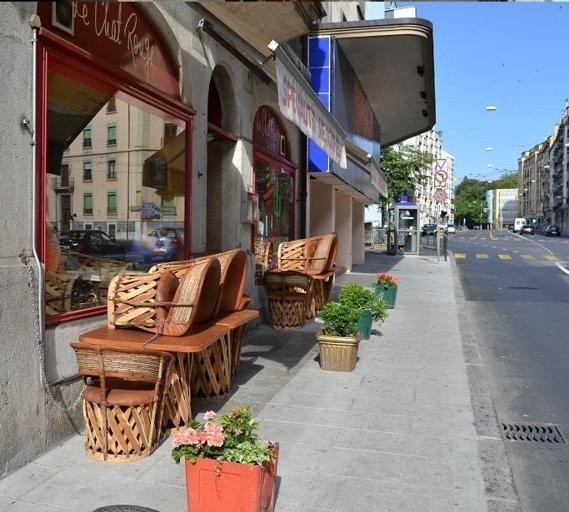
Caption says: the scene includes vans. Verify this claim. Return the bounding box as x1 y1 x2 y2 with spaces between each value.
513 217 526 233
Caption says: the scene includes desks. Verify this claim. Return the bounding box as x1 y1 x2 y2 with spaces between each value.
78 310 260 438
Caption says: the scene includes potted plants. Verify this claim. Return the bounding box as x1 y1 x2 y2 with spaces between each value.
314 301 364 372
338 282 389 341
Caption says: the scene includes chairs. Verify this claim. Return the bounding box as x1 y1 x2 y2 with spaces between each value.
254 235 282 271
106 248 248 337
69 341 168 462
45 250 134 315
263 232 345 329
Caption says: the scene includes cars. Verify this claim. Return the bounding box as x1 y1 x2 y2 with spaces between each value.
446 224 457 234
56 231 127 261
520 225 536 235
545 225 561 236
140 227 186 261
421 224 437 236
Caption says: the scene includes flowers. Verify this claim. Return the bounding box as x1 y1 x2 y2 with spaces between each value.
170 407 276 466
376 272 401 285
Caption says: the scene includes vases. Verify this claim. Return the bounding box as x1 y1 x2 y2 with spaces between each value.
371 282 398 309
184 439 280 512
